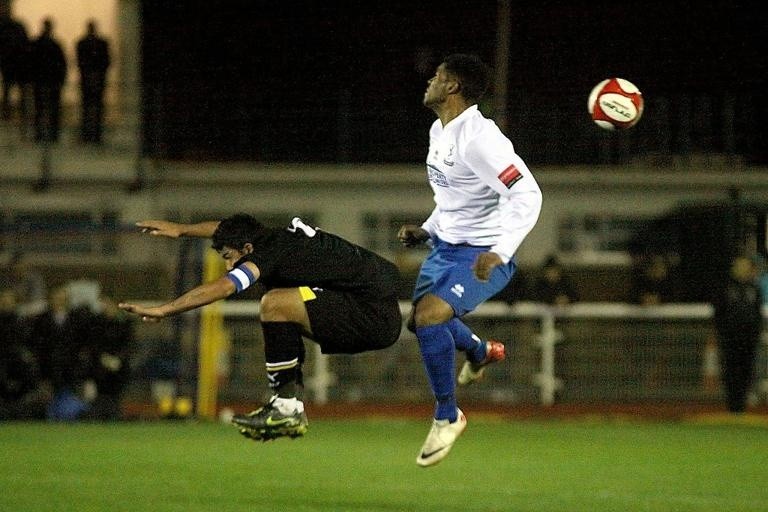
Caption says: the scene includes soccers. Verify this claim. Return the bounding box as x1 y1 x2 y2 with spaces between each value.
587 78 644 131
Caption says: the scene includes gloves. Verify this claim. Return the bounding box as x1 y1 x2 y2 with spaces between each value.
472 250 505 282
397 223 430 251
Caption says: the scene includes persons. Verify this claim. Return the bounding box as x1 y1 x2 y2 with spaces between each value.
29 16 67 146
147 310 199 420
629 204 766 413
484 258 579 389
1 249 129 423
397 51 544 472
0 0 27 125
119 214 404 443
74 17 112 146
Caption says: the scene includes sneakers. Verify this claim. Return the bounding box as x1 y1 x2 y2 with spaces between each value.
411 407 469 468
230 404 308 440
456 339 506 387
